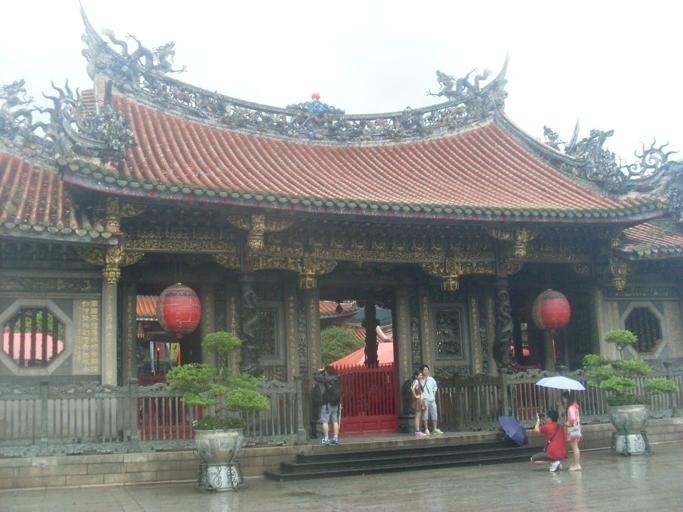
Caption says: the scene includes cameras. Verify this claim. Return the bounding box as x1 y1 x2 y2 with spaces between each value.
538 414 545 417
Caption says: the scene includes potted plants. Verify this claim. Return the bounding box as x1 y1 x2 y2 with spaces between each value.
166 330 270 493
583 329 679 456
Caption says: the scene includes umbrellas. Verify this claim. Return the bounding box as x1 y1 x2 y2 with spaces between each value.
536 375 586 404
497 415 527 446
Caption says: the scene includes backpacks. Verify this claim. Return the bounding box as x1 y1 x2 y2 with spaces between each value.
326 383 340 405
404 378 413 400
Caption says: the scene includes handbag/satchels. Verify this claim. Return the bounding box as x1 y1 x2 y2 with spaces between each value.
568 426 581 439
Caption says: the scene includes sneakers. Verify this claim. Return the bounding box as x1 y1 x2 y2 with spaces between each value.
322 436 339 444
568 463 581 471
549 461 563 471
415 431 427 437
425 430 430 436
432 429 443 435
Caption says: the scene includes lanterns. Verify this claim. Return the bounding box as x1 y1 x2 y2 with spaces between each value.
532 287 572 338
154 280 202 342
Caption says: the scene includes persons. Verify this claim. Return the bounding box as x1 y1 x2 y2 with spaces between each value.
313 365 340 445
419 364 444 436
556 391 583 471
410 370 427 438
530 410 567 472
